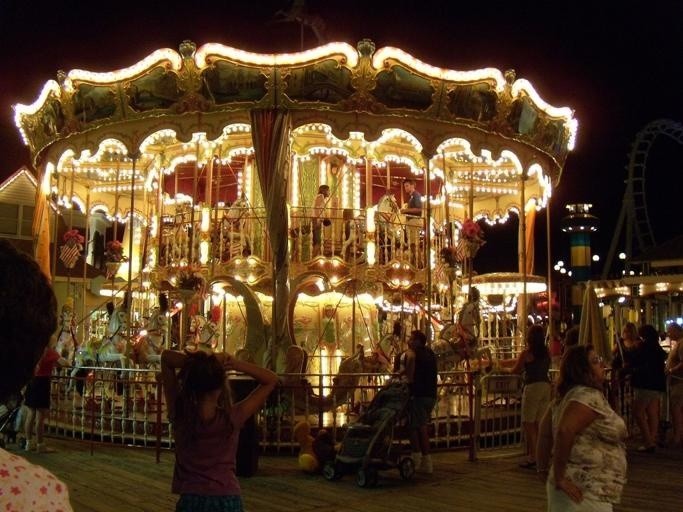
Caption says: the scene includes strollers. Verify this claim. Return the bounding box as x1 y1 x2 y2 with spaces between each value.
0 394 26 450
319 378 417 488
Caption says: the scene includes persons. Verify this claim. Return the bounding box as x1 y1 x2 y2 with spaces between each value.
400 179 423 269
312 185 330 258
161 351 279 512
539 344 628 510
394 319 438 474
1 237 73 512
502 324 551 467
527 317 682 450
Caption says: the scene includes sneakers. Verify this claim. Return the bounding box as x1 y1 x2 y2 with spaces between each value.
36 443 56 453
417 462 432 473
25 440 36 452
